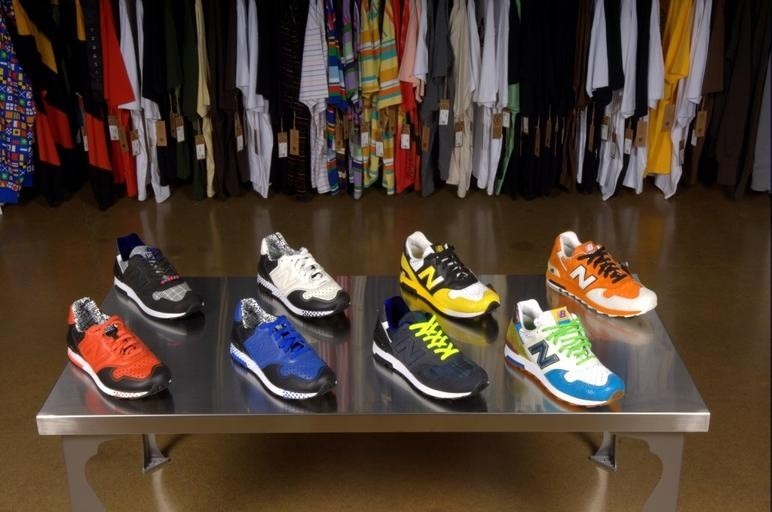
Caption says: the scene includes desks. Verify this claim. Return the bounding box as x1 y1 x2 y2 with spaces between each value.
35 276 712 472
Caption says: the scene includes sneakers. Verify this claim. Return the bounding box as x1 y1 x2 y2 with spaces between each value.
503 360 623 413
546 286 655 346
256 232 350 317
253 288 352 343
229 296 336 400
372 358 489 416
372 294 502 399
398 230 501 321
399 287 499 347
231 359 338 416
66 296 172 401
545 230 658 318
113 232 202 319
68 362 176 414
111 288 204 347
503 299 626 406
